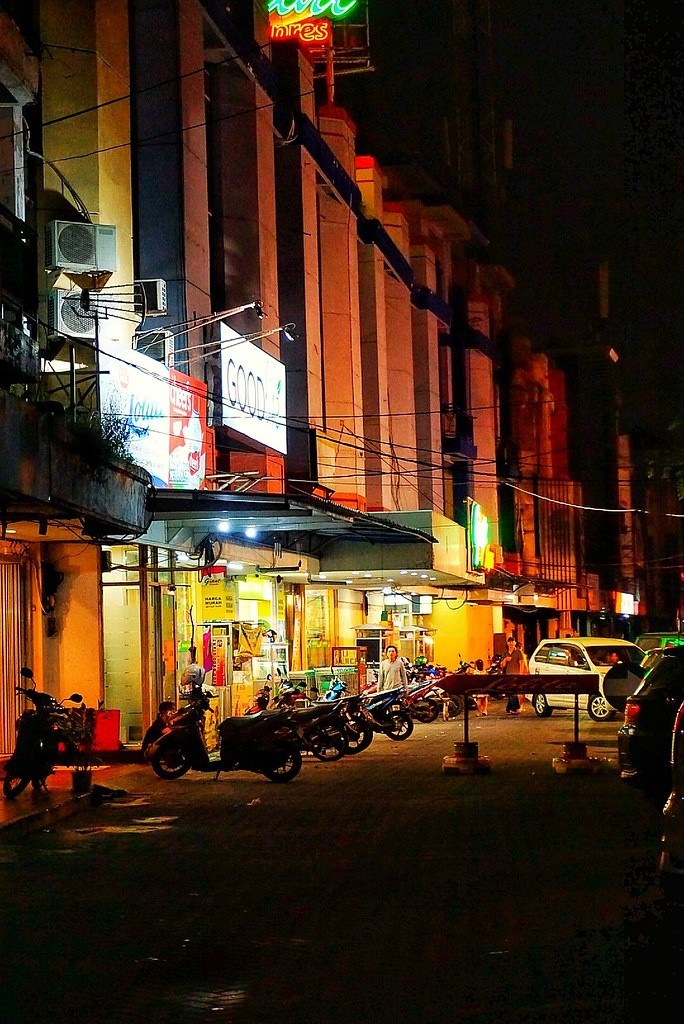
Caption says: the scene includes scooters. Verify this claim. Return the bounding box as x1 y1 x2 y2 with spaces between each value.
144 650 303 786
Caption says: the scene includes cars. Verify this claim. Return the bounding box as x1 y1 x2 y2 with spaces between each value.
636 631 684 654
615 653 684 785
656 699 683 891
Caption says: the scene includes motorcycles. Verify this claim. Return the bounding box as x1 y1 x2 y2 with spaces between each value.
2 666 88 802
233 652 502 763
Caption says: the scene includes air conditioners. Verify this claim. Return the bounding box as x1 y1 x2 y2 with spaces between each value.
43 220 119 274
46 288 123 341
134 278 168 317
132 329 175 370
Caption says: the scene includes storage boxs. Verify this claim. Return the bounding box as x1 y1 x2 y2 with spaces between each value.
253 640 290 711
331 646 369 697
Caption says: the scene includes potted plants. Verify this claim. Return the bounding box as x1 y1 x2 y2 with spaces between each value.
67 698 105 793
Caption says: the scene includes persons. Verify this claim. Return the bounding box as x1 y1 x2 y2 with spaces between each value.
607 651 619 665
514 642 529 714
472 657 490 717
498 637 525 715
378 645 408 732
140 701 177 764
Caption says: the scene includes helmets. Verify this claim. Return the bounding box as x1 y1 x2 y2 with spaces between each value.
182 665 206 687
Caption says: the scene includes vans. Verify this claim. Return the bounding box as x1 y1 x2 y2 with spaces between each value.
525 636 651 721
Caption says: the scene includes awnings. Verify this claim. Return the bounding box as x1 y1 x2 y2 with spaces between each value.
154 485 439 554
